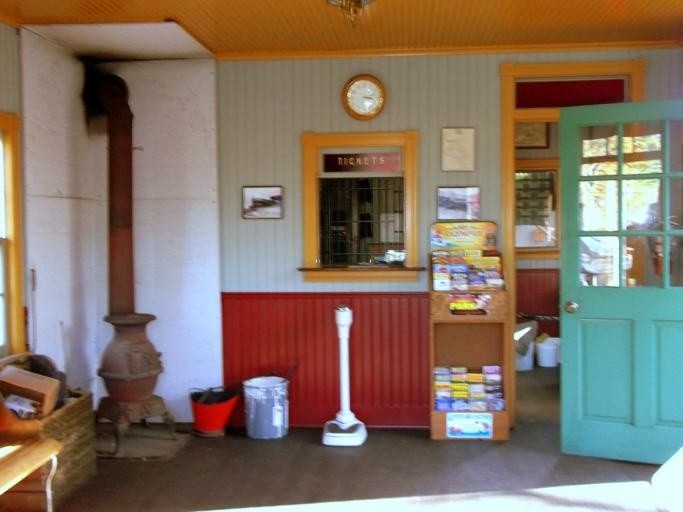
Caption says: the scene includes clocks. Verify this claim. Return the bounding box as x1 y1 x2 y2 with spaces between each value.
341 73 386 122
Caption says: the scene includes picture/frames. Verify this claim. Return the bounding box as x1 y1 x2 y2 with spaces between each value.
435 184 481 221
513 121 549 149
240 184 284 220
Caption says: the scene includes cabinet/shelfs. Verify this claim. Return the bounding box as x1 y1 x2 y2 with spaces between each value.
426 221 509 442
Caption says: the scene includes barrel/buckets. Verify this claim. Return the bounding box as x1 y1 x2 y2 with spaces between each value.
536 336 559 368
547 337 560 364
514 341 535 371
242 376 290 440
